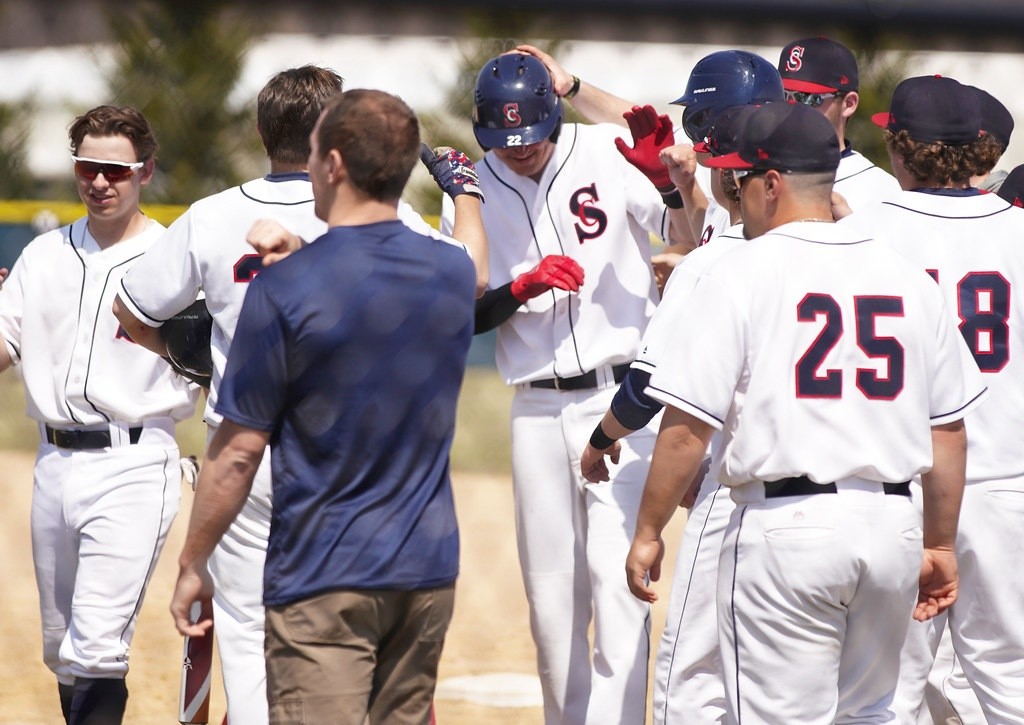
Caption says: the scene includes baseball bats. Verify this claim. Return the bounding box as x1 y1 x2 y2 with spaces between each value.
179 597 214 725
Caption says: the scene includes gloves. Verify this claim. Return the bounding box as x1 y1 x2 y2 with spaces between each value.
511 253 585 305
614 104 683 195
418 141 486 205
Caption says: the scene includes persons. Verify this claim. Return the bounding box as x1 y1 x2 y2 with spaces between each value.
504 36 900 725
112 64 490 724
626 100 990 725
836 74 1024 725
442 55 695 725
0 104 205 725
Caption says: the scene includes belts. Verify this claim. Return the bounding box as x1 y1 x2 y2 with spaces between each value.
764 479 911 499
530 361 631 390
45 424 143 450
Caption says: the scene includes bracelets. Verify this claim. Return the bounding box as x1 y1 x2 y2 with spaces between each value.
660 187 677 195
563 74 580 99
590 421 618 450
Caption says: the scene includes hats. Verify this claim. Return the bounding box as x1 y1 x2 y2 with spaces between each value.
871 74 983 146
996 163 1024 210
693 104 768 156
703 99 842 174
777 34 860 96
964 85 1015 155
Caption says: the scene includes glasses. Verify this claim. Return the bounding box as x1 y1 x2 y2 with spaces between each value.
722 167 794 194
70 153 144 182
784 89 844 108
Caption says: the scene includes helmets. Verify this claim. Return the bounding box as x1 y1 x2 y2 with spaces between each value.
470 53 564 153
664 49 786 143
162 297 214 388
